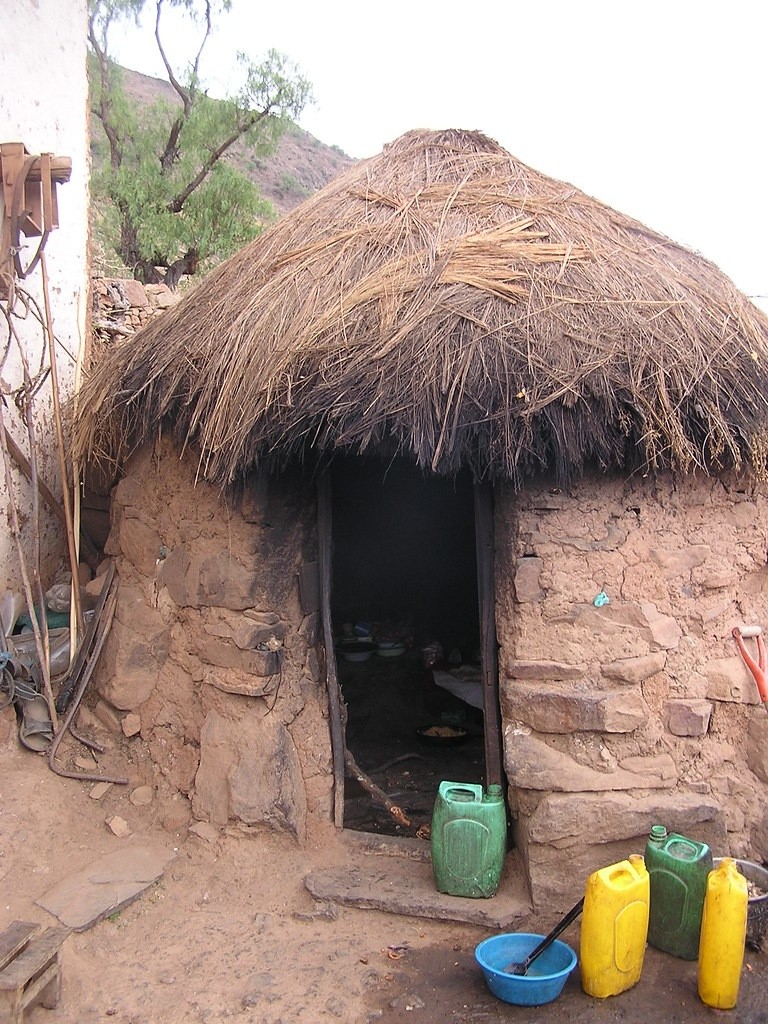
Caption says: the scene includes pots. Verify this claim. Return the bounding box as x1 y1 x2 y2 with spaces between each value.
712 856 768 944
414 721 473 747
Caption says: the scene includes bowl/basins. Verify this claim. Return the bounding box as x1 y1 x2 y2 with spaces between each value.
342 650 375 662
476 933 578 1006
378 642 408 656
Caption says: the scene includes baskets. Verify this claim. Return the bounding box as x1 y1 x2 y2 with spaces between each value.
415 722 468 747
712 858 768 945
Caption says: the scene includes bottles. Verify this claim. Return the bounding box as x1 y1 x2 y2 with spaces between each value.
579 854 650 998
45 583 99 613
431 780 507 900
697 857 748 1008
644 825 714 961
